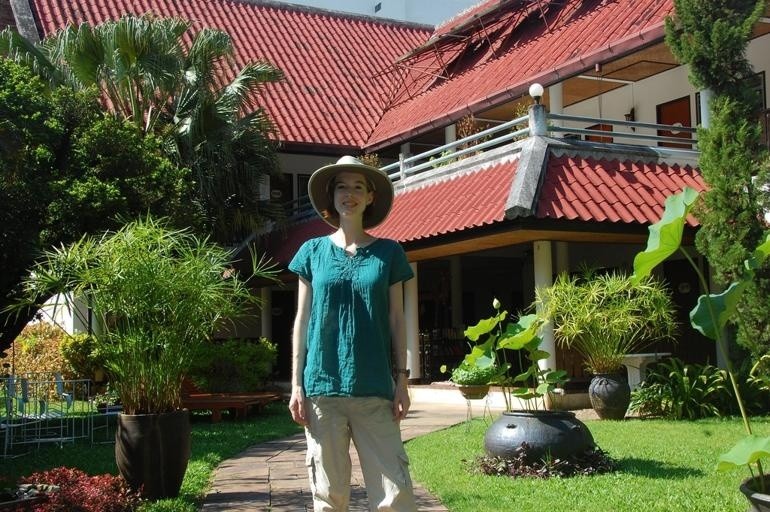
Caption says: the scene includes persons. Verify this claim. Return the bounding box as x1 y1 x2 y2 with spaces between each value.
286 156 421 510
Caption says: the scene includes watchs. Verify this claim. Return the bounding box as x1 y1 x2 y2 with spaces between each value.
396 367 410 380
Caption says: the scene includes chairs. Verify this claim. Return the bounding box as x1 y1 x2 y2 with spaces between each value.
0 372 123 459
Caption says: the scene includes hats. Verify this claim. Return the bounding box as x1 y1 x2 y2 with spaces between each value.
308 155 394 229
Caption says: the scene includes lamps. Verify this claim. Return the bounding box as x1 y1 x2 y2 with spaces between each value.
528 83 544 106
624 108 635 132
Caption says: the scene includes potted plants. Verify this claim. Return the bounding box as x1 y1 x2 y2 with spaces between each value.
0 211 284 500
630 185 770 512
649 357 727 419
451 349 507 399
464 298 600 473
522 267 680 419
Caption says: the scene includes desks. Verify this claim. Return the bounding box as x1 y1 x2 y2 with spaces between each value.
420 339 456 381
